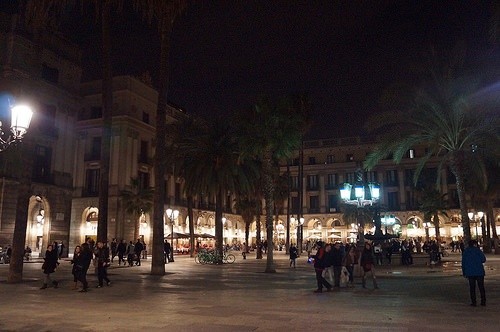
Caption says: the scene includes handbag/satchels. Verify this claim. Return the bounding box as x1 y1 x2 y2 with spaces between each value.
341 266 350 283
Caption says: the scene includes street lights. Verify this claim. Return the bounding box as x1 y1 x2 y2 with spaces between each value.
338 183 382 268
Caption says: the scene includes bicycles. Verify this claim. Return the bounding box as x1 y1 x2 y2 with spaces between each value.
194 245 236 264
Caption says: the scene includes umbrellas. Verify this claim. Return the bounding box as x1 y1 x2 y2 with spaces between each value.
165 232 215 239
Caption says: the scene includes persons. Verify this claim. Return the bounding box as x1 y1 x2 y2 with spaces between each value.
306 239 380 293
39 245 59 289
450 236 483 252
70 237 111 292
164 240 171 264
289 243 301 269
110 238 146 267
25 246 31 261
462 240 487 307
373 237 448 266
52 240 65 271
198 239 286 260
0 244 12 264
